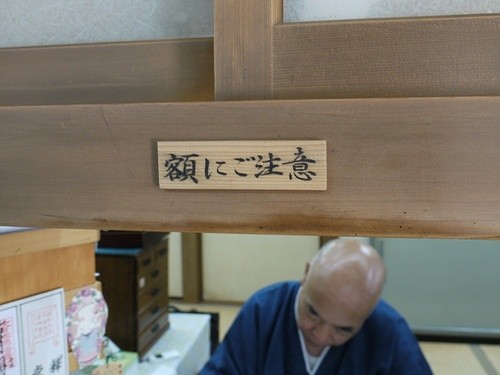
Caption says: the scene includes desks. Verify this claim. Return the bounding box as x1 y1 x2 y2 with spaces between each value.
120 311 219 375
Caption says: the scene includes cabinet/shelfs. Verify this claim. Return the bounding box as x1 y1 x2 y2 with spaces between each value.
97 239 169 356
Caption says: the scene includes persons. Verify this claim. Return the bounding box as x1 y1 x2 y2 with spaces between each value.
196 237 437 375
